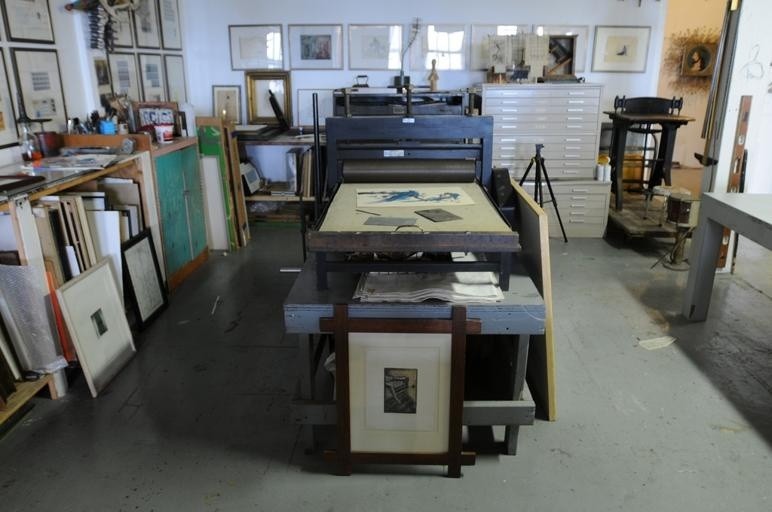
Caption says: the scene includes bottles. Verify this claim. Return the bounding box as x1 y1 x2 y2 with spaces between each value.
19 121 43 162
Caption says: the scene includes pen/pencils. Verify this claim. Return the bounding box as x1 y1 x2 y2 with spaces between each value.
105 110 114 121
90 110 99 128
356 209 380 217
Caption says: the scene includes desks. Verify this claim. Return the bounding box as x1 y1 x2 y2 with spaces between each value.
1 153 151 439
230 126 329 251
685 190 771 323
604 108 695 212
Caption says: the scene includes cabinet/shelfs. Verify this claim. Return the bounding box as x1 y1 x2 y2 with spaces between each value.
156 140 213 281
519 178 610 239
483 85 601 181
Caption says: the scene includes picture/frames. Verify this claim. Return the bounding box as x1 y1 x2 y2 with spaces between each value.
320 299 480 479
296 88 335 128
680 41 719 77
106 0 189 108
245 70 292 124
51 226 169 402
0 0 68 151
211 85 243 127
